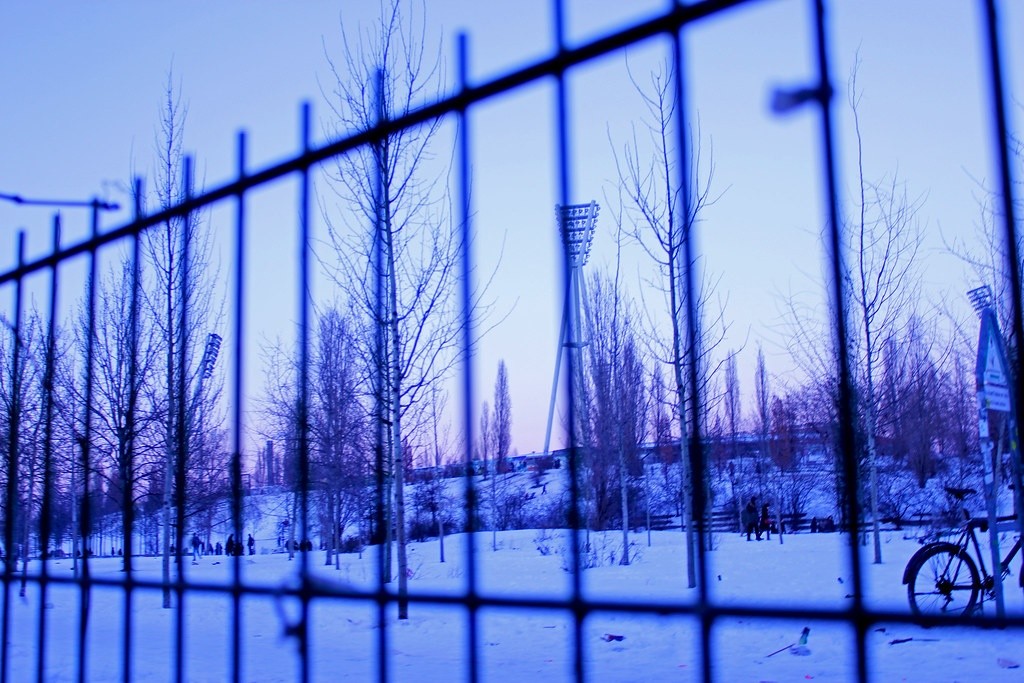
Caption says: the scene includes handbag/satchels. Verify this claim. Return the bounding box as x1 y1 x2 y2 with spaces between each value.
765 519 772 524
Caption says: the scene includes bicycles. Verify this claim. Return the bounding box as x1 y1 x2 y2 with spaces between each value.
902 485 1024 628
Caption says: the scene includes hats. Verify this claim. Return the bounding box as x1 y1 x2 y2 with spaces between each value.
750 496 758 504
765 503 772 507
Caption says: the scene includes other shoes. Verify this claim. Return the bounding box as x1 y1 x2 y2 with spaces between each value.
747 538 753 541
758 538 764 541
756 537 762 540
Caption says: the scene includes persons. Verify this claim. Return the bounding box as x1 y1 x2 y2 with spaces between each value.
192 531 255 561
170 544 175 555
285 537 313 551
740 496 777 541
809 517 817 533
